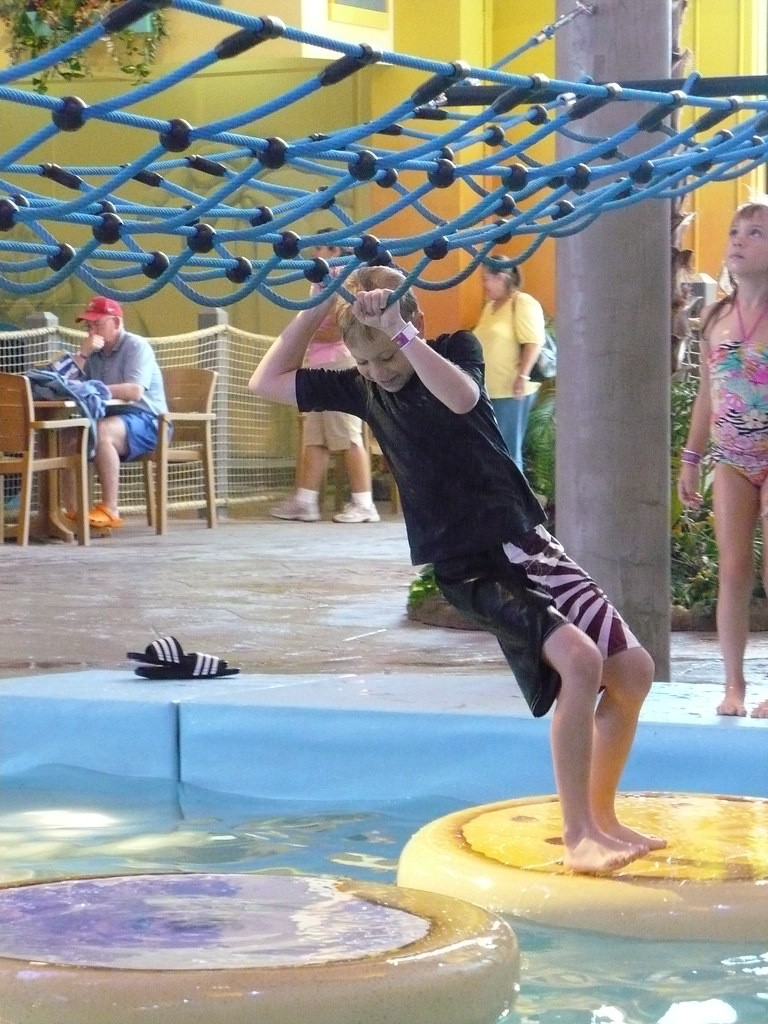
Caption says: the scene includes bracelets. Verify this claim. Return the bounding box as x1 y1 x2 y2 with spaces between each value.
391 321 419 349
681 450 703 466
76 351 89 359
518 373 531 380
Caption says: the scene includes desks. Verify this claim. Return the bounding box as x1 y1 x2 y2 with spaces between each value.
4 398 134 544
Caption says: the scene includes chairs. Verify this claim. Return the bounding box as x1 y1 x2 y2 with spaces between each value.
87 365 220 534
0 373 91 546
294 414 402 515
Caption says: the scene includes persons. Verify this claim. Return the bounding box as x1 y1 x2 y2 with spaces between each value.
679 205 768 718
247 259 667 872
271 228 381 523
473 255 544 473
62 298 175 528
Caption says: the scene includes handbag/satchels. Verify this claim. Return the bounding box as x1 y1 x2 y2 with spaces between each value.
512 291 558 382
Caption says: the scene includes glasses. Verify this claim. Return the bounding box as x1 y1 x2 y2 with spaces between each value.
81 317 116 331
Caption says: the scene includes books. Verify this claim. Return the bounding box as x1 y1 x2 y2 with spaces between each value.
36 354 86 380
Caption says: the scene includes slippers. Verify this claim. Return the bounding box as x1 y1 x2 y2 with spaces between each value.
134 651 241 680
87 504 124 528
126 635 193 667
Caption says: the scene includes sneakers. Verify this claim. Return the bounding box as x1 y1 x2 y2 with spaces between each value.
267 496 321 521
331 501 381 523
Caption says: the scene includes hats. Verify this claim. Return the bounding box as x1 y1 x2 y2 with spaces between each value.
74 294 123 323
